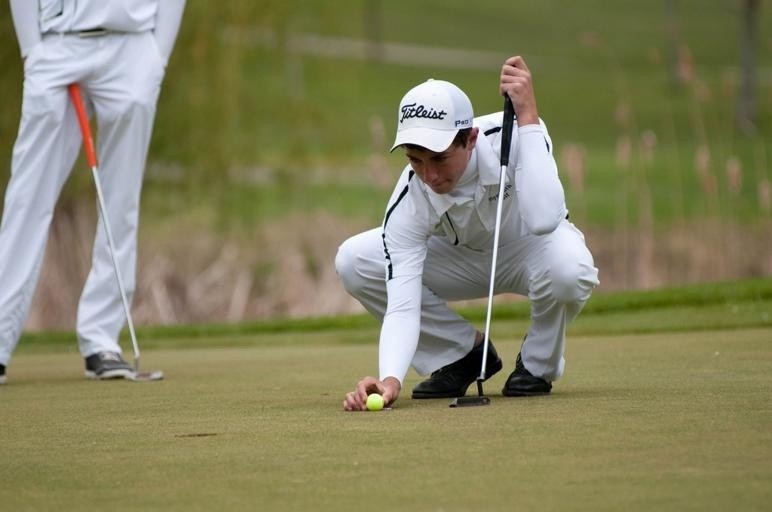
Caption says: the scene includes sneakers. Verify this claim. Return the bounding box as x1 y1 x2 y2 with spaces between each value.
85 351 136 382
0 364 10 388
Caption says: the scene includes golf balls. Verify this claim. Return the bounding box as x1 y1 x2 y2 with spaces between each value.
367 394 384 411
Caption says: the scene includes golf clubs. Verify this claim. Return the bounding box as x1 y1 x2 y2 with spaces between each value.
69 83 165 380
448 93 513 410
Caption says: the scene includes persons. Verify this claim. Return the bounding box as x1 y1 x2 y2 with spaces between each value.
336 56 598 411
2 0 189 379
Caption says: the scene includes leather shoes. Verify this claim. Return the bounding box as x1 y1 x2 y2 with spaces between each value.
411 331 504 401
501 332 554 398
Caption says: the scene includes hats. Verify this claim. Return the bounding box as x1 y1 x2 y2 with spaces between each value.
389 77 475 155
